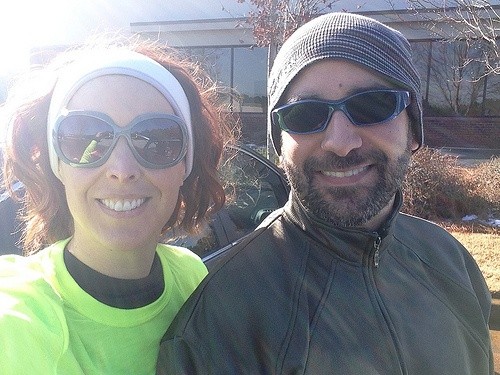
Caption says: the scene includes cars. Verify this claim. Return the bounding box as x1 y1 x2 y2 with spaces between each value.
0 132 291 265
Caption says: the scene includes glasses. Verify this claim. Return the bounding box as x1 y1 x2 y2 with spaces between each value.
271 90 412 135
51 110 190 170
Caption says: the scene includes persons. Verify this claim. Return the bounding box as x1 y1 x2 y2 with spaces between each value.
155 11 493 375
0 31 243 375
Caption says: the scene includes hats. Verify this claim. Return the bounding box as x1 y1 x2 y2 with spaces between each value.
267 13 424 160
